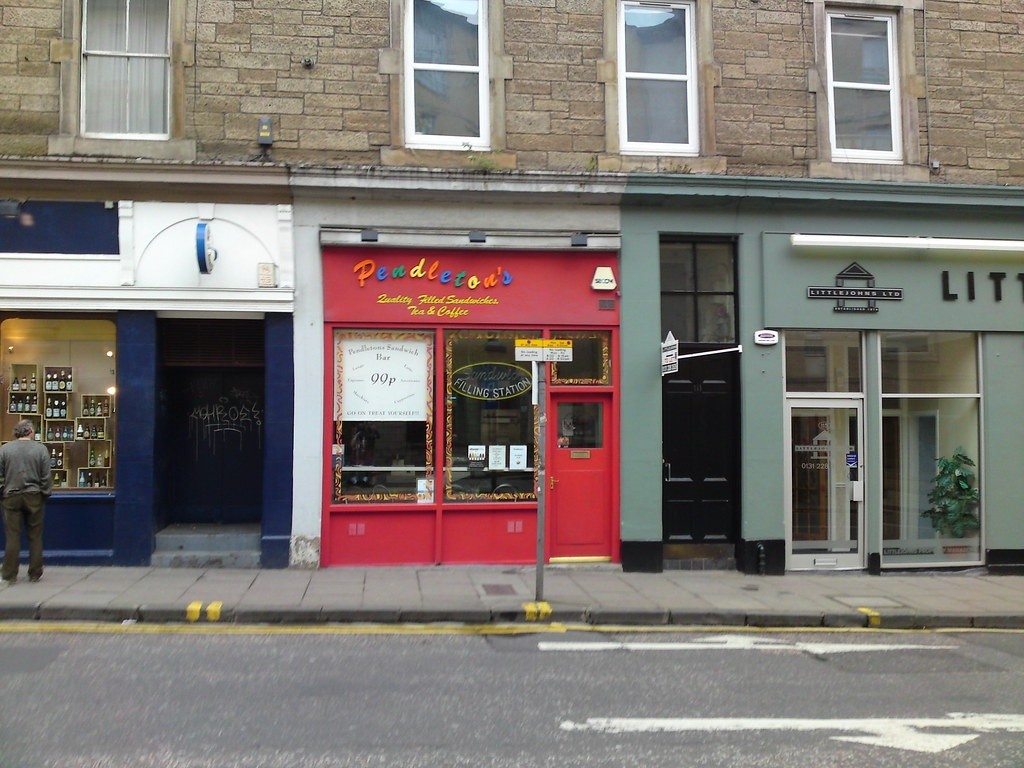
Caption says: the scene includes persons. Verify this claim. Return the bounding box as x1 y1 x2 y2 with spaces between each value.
0 419 53 583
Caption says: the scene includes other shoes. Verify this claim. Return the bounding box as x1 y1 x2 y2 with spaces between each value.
30 573 39 582
7 578 17 584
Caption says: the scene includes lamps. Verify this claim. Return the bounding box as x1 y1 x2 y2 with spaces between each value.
257 116 274 145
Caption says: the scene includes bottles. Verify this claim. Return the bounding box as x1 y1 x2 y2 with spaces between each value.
45 369 72 391
90 447 109 467
77 423 104 439
10 393 37 413
79 471 106 487
53 472 66 487
13 373 36 391
34 421 41 441
349 429 373 487
83 398 109 416
46 396 66 418
48 424 73 441
50 448 62 469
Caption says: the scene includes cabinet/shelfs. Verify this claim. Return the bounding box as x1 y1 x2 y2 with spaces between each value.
7 361 116 491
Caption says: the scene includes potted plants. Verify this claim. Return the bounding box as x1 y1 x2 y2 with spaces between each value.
922 445 981 558
